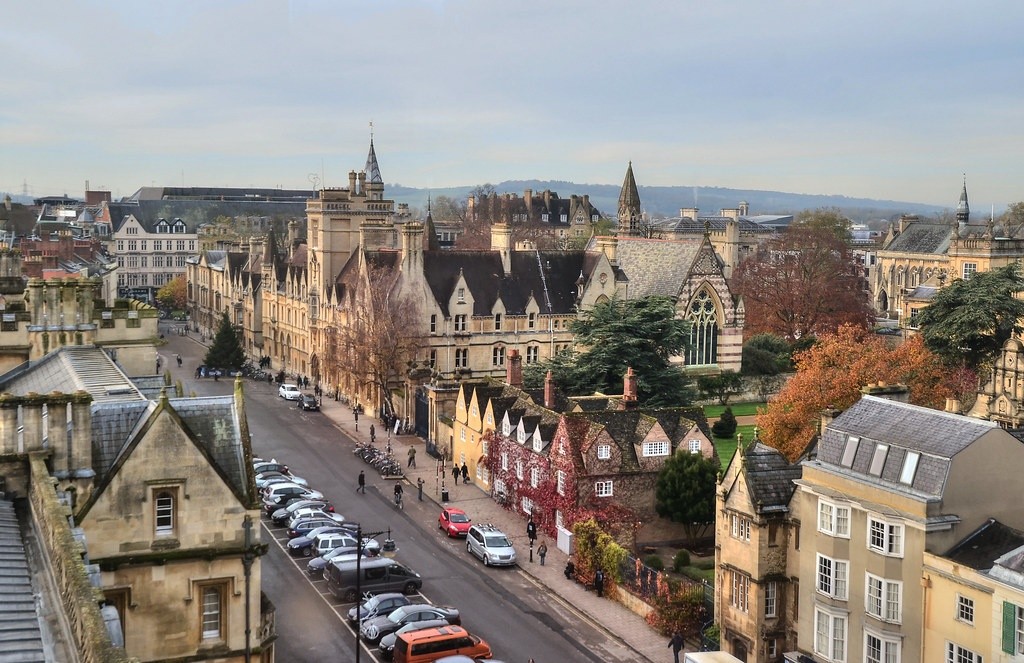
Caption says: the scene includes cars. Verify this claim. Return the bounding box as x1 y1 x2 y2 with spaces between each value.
253 455 423 603
347 592 506 663
296 393 320 412
278 384 302 401
438 508 472 538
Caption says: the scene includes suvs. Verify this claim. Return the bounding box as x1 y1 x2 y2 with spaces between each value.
466 523 517 568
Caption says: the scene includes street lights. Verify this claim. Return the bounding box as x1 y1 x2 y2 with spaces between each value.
355 523 400 663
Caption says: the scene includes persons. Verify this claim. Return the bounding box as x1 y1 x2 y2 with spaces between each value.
157 320 202 379
460 462 468 484
259 355 319 397
357 470 366 494
668 631 684 663
536 540 547 566
417 477 426 501
370 413 395 442
595 570 604 597
563 559 574 579
407 445 417 468
451 463 460 485
394 481 403 507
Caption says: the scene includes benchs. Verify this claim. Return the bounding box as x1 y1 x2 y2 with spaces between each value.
571 565 595 592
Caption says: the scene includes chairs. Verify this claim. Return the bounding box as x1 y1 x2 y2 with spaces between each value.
460 515 465 520
426 645 432 652
415 645 422 652
452 515 456 520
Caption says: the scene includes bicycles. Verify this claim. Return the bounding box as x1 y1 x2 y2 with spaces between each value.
247 369 267 381
352 441 402 476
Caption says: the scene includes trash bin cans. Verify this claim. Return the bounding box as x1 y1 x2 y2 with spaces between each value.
442 491 449 501
